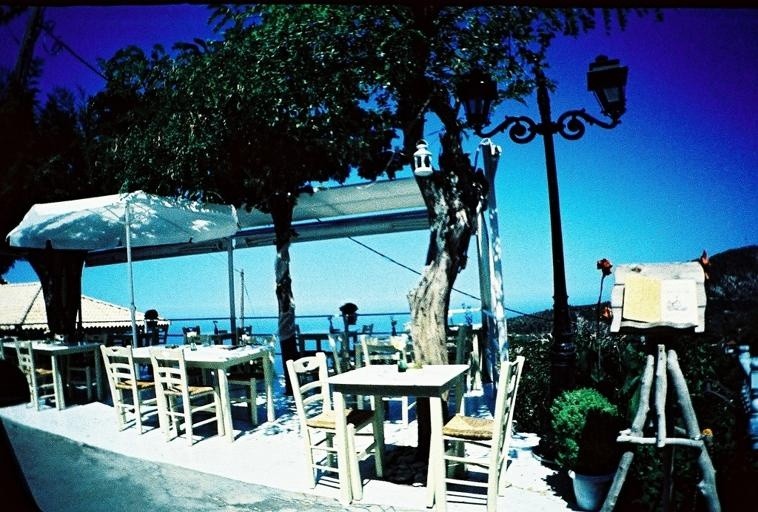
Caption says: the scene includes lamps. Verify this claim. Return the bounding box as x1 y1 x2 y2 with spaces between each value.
413 139 433 176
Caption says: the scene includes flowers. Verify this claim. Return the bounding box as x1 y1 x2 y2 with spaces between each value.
588 256 616 382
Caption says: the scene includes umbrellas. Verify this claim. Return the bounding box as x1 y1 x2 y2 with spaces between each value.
4 188 243 417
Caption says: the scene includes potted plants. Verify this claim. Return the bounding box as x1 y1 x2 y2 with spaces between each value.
549 383 628 510
